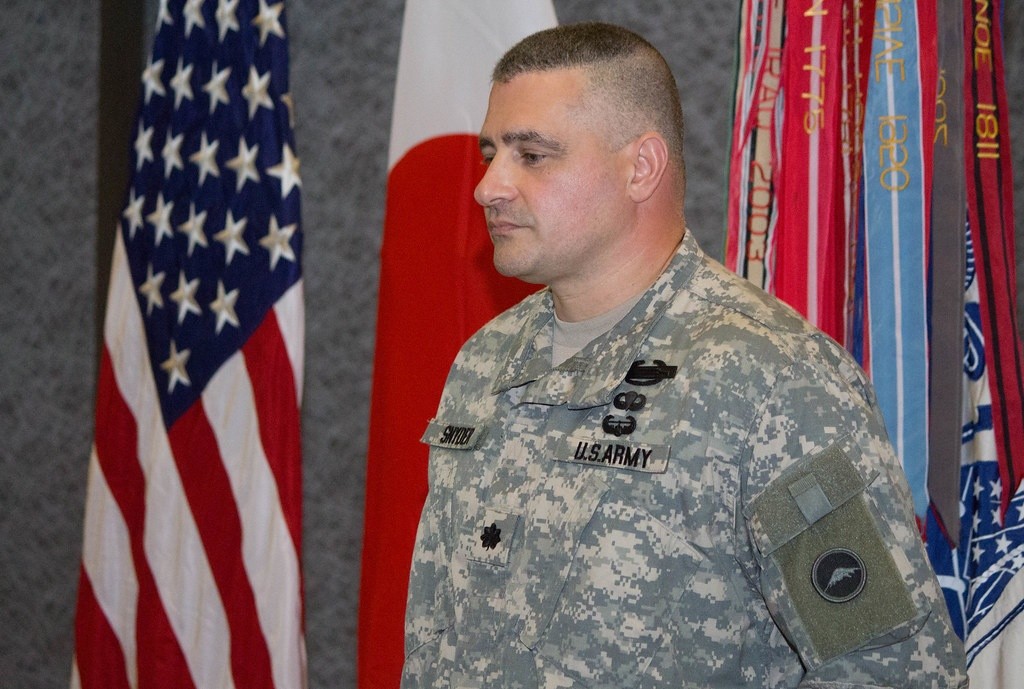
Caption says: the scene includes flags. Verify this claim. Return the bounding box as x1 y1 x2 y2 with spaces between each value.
357 0 559 688
70 1 306 688
724 0 1024 688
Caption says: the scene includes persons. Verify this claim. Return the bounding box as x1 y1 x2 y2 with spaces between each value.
400 22 969 688
